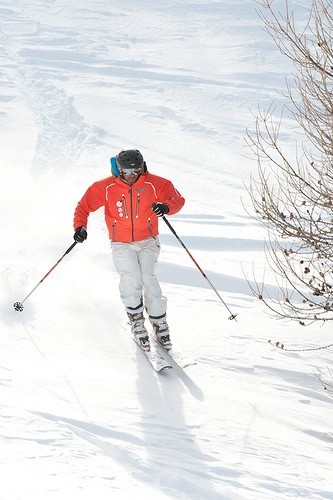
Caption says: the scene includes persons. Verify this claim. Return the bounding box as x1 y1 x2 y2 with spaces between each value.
74 149 185 350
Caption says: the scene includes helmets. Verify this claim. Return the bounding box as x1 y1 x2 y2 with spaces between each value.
117 150 144 169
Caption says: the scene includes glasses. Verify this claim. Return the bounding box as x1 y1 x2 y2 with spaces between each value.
119 168 142 176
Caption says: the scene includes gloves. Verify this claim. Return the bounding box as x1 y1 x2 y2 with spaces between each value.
151 201 169 217
73 227 87 243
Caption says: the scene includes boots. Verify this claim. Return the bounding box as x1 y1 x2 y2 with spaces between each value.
126 305 150 351
149 311 173 352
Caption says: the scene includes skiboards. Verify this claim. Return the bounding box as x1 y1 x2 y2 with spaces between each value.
120 319 173 373
150 328 198 369
